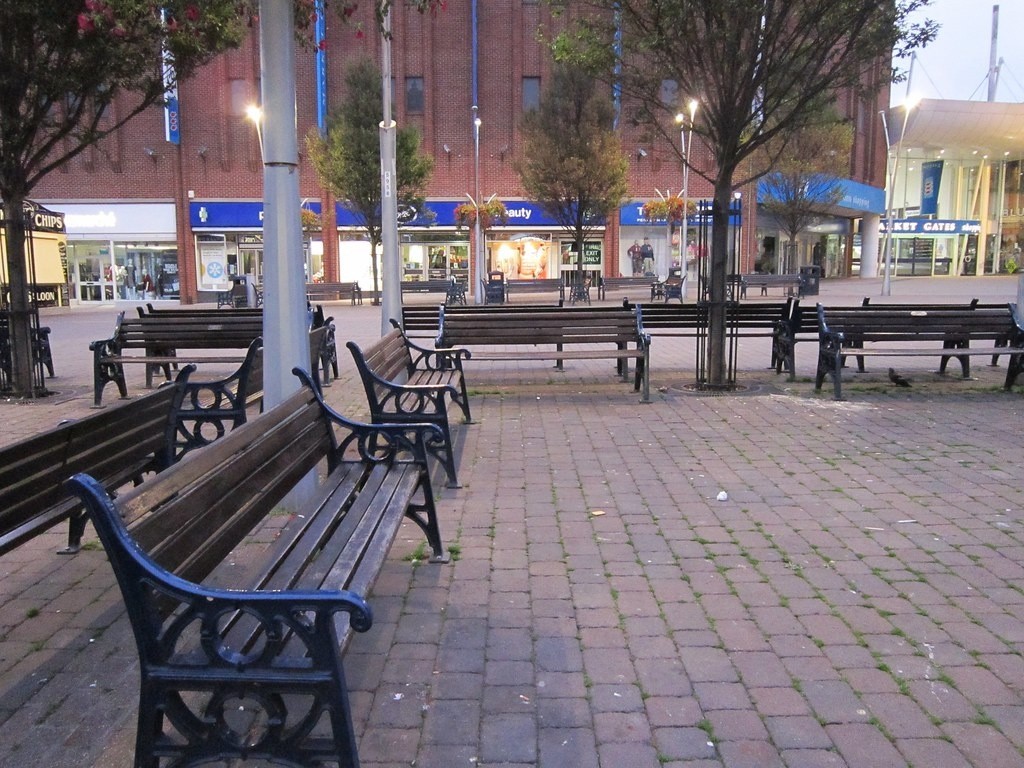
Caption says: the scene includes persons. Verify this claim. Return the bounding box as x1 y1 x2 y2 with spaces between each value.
125 258 163 300
628 237 655 276
813 242 823 274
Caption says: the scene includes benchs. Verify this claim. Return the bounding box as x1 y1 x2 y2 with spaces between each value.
0 276 1024 768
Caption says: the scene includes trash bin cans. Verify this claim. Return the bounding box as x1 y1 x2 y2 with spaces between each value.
667 266 681 279
487 269 505 305
231 274 256 309
799 265 821 296
